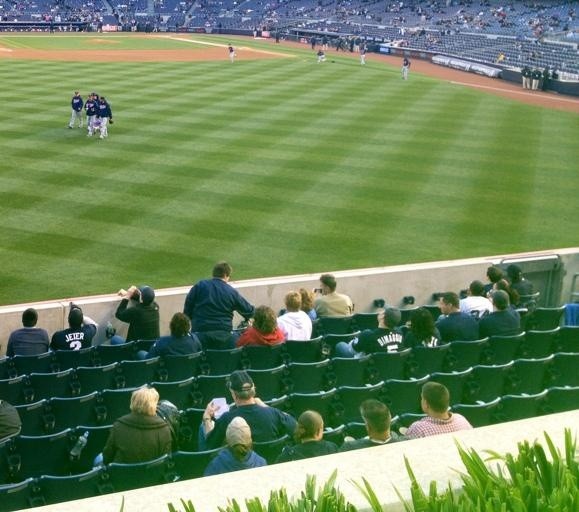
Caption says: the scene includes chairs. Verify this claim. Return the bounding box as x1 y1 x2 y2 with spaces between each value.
0 0 579 89
0 285 579 511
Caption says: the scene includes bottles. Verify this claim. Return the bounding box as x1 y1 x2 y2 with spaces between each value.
69 430 90 457
106 321 114 334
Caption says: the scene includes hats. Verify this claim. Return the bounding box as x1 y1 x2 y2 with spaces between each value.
68 305 83 326
226 369 254 392
225 416 252 448
138 285 155 303
503 264 523 277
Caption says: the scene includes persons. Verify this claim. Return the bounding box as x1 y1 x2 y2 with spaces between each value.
204 416 266 476
0 0 579 90
110 285 160 344
341 399 409 452
235 265 537 358
407 382 473 438
136 312 202 360
6 308 49 357
198 371 297 452
68 92 113 139
275 411 339 463
50 308 99 351
184 263 254 349
103 387 171 465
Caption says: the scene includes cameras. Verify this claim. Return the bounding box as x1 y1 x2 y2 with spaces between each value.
314 288 322 294
